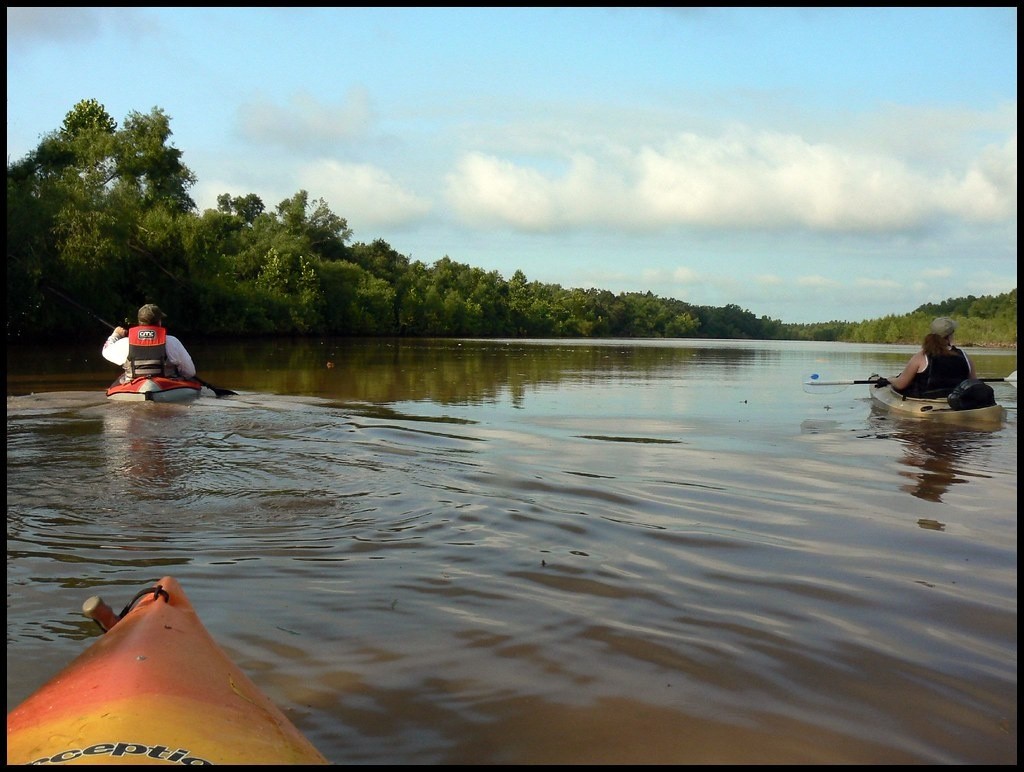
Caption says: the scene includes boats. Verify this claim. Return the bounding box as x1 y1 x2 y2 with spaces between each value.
106 375 203 402
869 371 1008 425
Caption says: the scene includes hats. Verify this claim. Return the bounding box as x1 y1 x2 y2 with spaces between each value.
138 304 167 324
931 317 957 337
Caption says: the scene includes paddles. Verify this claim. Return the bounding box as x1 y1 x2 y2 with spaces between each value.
45 285 239 398
801 368 1017 396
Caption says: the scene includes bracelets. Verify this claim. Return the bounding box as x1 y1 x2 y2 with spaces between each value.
887 375 897 381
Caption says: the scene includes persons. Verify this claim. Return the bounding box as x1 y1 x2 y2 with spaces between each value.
887 317 977 399
102 304 197 383
